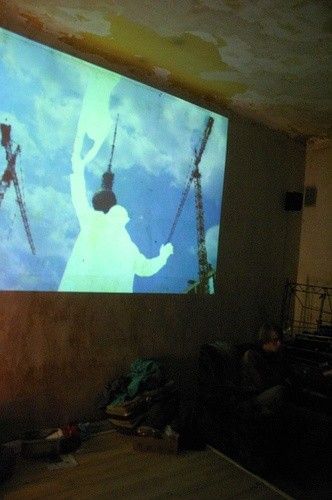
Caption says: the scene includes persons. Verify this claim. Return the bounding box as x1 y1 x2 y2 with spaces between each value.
55 156 174 293
73 57 121 171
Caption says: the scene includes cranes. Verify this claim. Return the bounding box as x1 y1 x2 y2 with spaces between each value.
0 120 39 258
164 115 216 296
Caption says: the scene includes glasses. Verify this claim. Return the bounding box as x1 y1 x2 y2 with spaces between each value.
268 336 283 343
